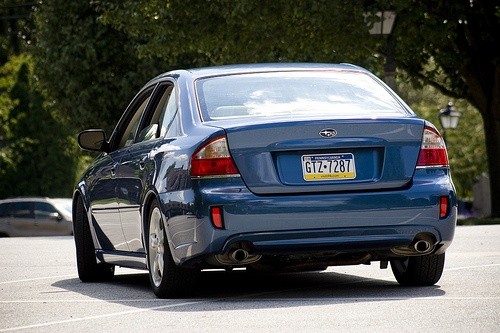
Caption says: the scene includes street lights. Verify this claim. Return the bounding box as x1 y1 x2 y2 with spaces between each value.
438 102 462 146
361 10 397 36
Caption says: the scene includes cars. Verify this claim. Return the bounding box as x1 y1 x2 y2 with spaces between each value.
71 62 457 299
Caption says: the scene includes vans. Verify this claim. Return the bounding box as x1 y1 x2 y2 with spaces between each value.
0 198 73 238
